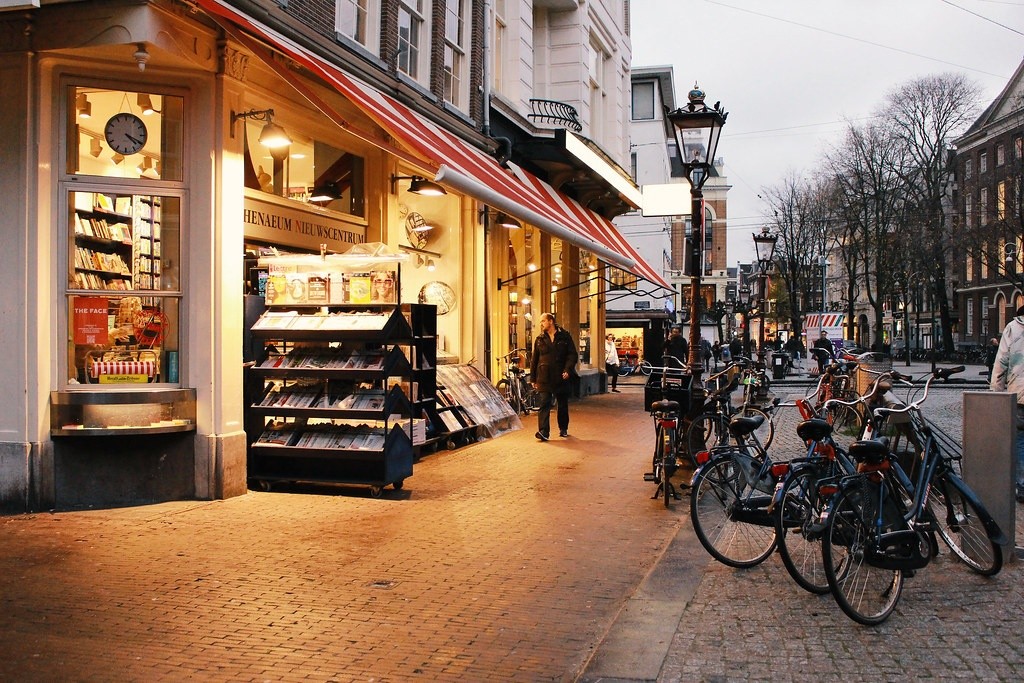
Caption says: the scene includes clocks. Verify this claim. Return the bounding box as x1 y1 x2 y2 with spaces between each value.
105 113 148 154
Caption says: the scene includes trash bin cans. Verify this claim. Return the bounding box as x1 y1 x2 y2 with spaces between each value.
721 344 732 361
854 352 892 436
766 348 777 369
771 353 789 379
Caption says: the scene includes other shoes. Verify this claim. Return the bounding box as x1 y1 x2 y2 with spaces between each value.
535 431 548 441
1015 481 1024 504
612 389 621 392
562 431 567 437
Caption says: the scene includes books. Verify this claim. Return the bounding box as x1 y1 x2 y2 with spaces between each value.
257 272 388 450
73 192 160 307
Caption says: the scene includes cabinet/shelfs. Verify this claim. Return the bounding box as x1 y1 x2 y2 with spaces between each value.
70 191 163 309
244 302 442 494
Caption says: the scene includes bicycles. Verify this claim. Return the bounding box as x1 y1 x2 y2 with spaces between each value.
617 353 654 377
810 367 1008 626
496 348 558 418
642 337 1000 596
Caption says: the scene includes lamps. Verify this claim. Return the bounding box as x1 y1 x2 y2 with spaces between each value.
478 208 522 228
230 108 293 148
390 174 447 196
307 188 335 201
134 43 151 72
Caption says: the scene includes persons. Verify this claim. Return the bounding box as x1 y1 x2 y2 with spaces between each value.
814 331 834 372
990 315 1024 506
664 328 688 368
372 273 394 303
712 341 721 370
702 338 712 371
605 334 621 393
729 337 742 361
530 313 578 441
767 336 794 351
986 338 999 383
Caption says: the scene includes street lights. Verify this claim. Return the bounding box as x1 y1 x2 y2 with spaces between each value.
738 282 753 385
662 78 729 458
751 223 779 402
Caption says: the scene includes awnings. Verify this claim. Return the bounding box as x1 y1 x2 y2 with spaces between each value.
200 0 679 294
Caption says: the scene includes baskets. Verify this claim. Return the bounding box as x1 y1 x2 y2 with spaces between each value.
644 371 694 415
